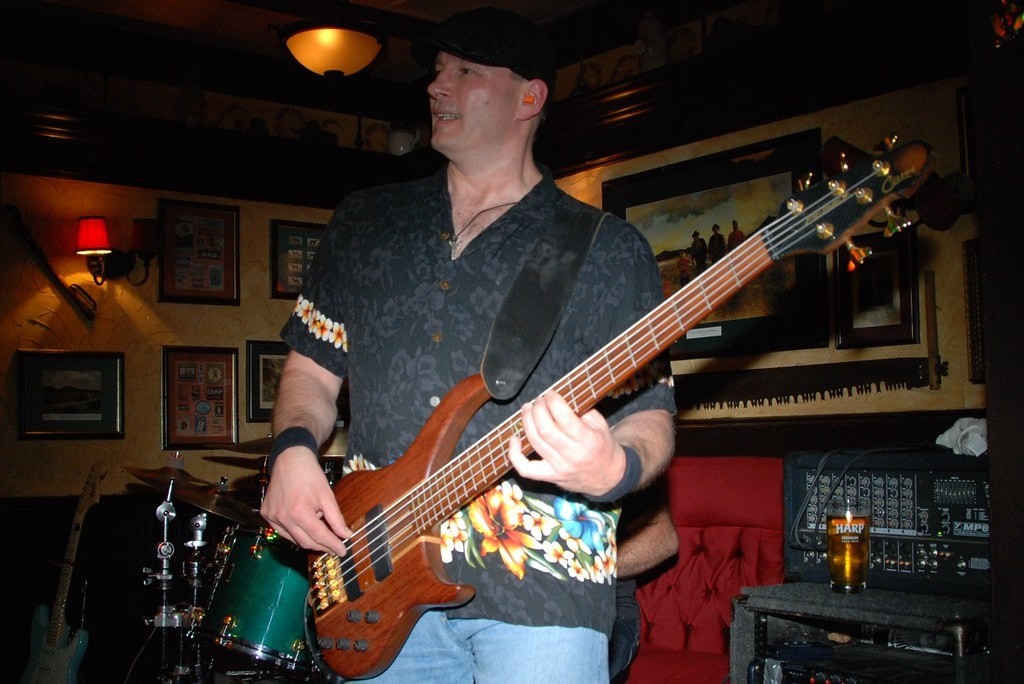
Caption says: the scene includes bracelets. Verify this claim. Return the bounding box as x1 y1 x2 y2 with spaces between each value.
581 446 640 505
267 426 319 477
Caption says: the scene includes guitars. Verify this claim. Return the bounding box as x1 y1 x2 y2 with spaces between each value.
18 460 109 684
307 133 938 681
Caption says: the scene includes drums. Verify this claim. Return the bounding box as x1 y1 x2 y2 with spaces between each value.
186 527 315 677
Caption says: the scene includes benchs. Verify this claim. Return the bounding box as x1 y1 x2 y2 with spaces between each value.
617 455 788 684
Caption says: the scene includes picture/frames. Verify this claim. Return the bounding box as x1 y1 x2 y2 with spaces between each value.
245 339 350 424
269 218 328 300
157 198 241 306
602 127 830 360
833 228 921 350
16 349 125 440
160 345 239 451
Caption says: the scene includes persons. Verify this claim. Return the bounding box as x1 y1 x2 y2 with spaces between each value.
257 7 677 684
606 486 678 680
678 219 746 279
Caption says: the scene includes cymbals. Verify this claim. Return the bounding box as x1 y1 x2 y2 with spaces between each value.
133 473 260 524
195 438 274 455
121 466 214 485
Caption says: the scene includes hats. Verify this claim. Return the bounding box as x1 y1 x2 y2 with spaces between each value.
410 7 548 84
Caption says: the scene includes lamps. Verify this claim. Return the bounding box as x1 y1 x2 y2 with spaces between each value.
72 215 163 287
264 14 388 78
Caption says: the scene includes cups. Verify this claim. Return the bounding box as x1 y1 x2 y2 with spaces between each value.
825 497 873 594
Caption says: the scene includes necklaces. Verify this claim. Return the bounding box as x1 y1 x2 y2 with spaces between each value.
448 201 519 247
